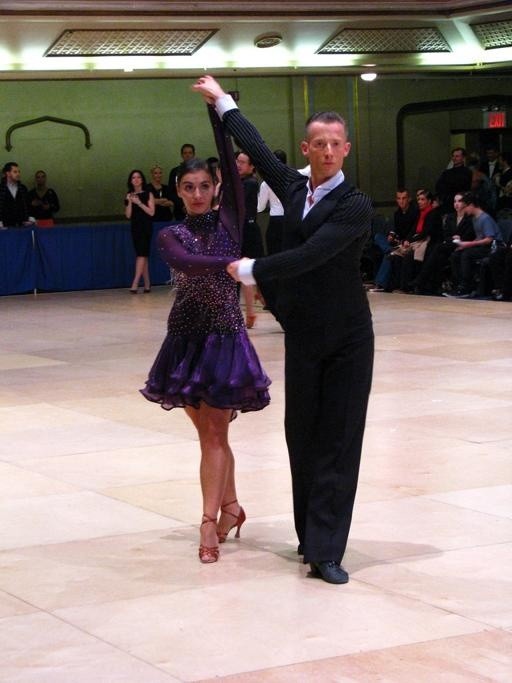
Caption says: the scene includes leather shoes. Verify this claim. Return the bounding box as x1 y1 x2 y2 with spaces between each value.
129 282 153 293
308 554 352 584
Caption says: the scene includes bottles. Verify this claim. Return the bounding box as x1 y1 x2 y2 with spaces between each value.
490 239 498 254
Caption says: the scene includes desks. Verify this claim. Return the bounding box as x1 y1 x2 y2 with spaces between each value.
1 220 178 294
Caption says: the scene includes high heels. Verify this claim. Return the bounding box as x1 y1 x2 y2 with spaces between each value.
197 513 221 563
216 499 246 542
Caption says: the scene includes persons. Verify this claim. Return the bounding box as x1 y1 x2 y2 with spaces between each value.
124 170 156 293
192 73 374 584
147 143 287 328
28 170 60 227
139 83 273 563
367 145 511 301
0 162 34 230
